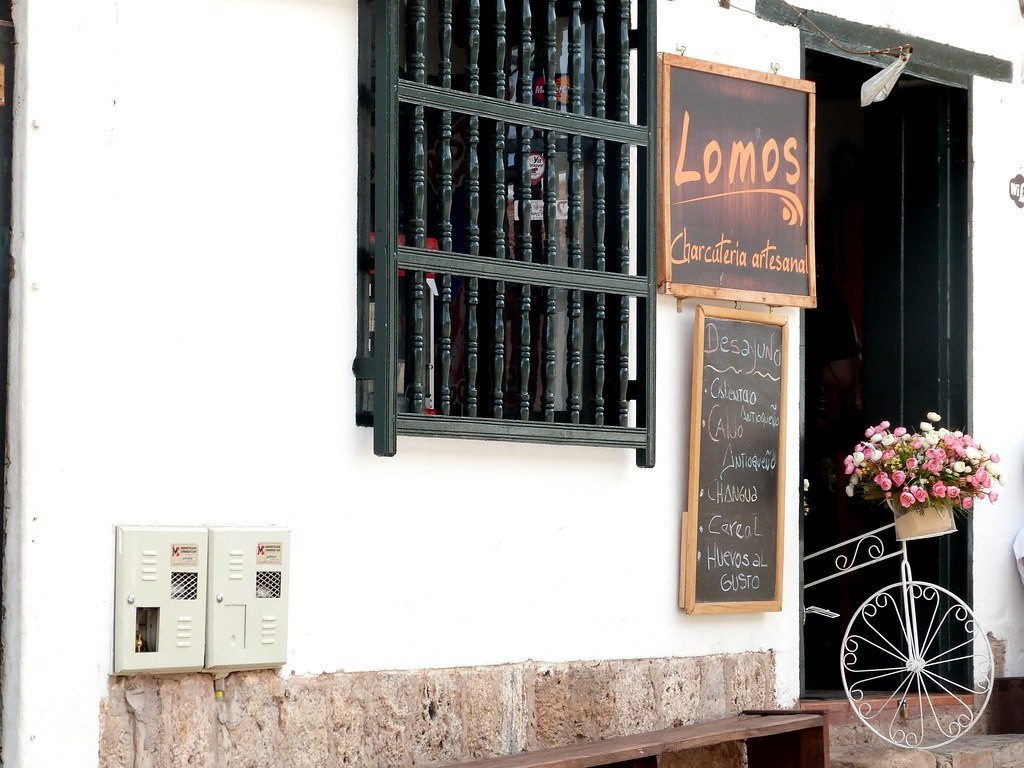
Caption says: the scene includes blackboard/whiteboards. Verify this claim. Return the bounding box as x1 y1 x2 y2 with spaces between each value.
677 304 789 613
656 52 817 308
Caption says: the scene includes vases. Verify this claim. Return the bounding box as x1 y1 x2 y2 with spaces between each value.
891 493 958 541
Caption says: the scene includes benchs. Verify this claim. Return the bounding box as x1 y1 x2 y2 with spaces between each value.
439 710 832 768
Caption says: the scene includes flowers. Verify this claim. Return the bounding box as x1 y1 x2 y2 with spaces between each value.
845 412 1006 522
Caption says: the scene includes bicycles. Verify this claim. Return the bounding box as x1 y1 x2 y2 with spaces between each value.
802 493 996 750
